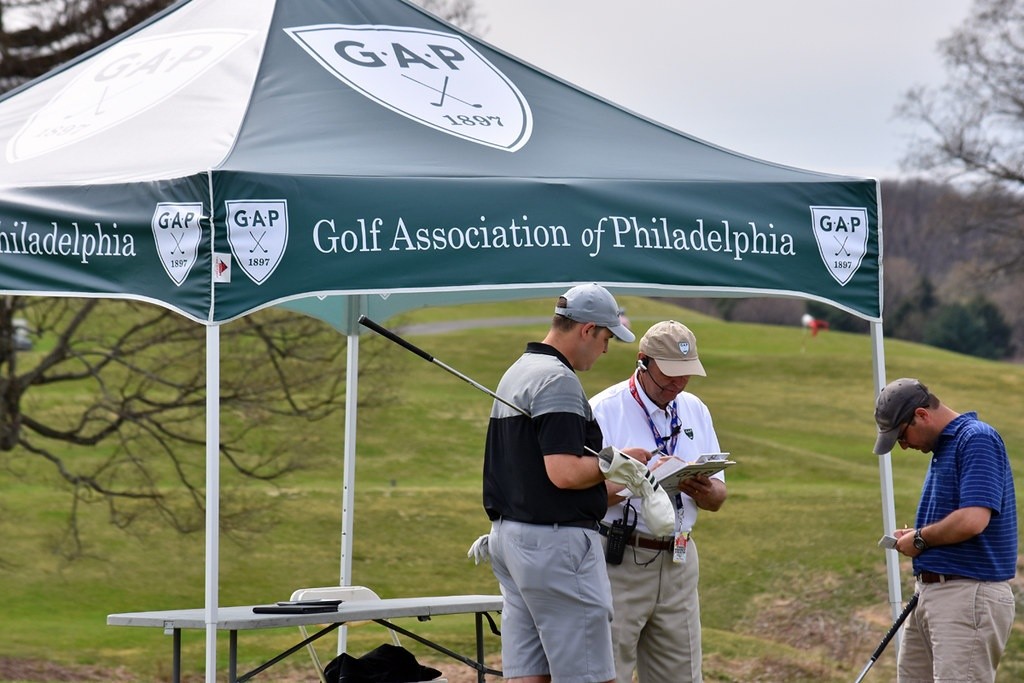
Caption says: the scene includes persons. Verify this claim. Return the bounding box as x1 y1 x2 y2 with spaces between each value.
588 321 727 683
482 284 653 683
872 378 1018 683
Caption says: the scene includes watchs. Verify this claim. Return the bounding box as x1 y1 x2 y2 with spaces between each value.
913 527 929 551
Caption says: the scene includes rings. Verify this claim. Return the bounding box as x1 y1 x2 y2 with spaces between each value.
694 490 698 495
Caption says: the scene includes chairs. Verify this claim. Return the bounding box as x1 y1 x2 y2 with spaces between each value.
290 586 448 683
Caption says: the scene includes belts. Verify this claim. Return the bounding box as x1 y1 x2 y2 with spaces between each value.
599 523 690 553
490 510 599 531
916 569 970 584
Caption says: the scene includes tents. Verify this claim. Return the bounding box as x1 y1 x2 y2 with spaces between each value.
0 0 907 683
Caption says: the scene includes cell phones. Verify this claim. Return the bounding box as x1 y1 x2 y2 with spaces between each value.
277 598 342 606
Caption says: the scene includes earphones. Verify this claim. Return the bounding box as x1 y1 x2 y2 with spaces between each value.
638 358 649 371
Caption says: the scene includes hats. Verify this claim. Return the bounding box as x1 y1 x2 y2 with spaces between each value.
871 378 931 455
639 319 707 377
556 283 635 342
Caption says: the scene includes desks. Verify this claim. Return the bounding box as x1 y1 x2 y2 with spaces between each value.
107 595 503 683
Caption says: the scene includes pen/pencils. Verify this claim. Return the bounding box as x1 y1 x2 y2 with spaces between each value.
658 451 669 457
905 524 908 529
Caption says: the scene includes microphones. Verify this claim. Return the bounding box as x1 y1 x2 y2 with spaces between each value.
647 369 664 391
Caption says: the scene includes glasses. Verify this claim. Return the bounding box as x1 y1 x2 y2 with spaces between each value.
897 405 930 442
